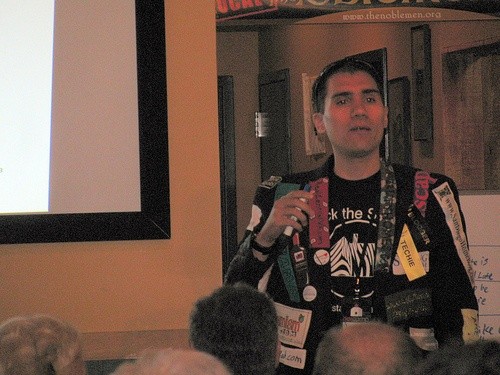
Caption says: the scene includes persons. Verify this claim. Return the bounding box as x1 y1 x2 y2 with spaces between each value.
0 283 500 375
224 57 480 354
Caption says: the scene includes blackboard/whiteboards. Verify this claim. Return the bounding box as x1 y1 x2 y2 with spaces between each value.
457 189 500 342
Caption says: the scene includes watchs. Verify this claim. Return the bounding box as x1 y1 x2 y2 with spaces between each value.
251 234 272 255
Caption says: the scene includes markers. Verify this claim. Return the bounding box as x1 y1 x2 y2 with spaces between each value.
278 198 307 240
